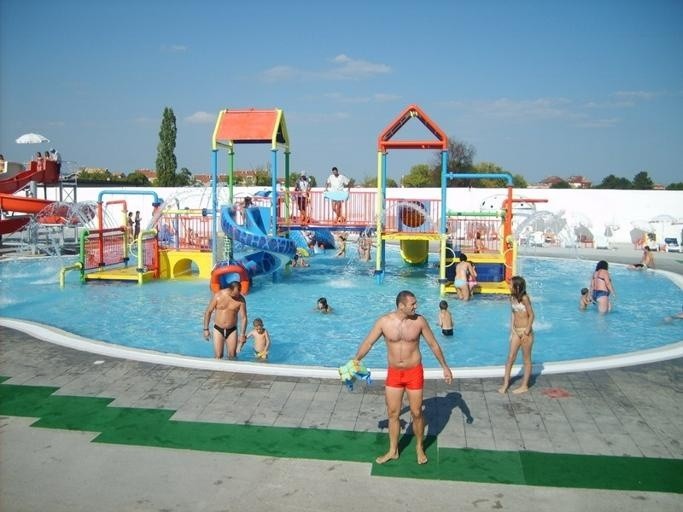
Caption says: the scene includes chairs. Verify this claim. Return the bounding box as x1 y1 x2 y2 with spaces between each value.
514 219 682 256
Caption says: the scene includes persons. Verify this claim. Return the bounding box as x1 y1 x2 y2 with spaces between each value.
235 317 271 361
35 150 61 170
315 298 331 313
158 224 171 241
454 253 477 300
202 281 246 361
590 259 616 316
625 245 656 272
496 275 535 394
324 167 351 222
577 287 593 312
128 211 142 240
436 299 453 338
294 171 312 224
472 232 484 254
291 230 371 266
0 154 5 172
350 290 454 466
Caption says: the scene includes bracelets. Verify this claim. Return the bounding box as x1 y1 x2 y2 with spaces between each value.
522 332 529 337
203 328 208 330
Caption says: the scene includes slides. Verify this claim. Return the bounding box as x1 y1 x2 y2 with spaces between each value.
215 200 294 291
0 159 92 236
397 204 429 267
